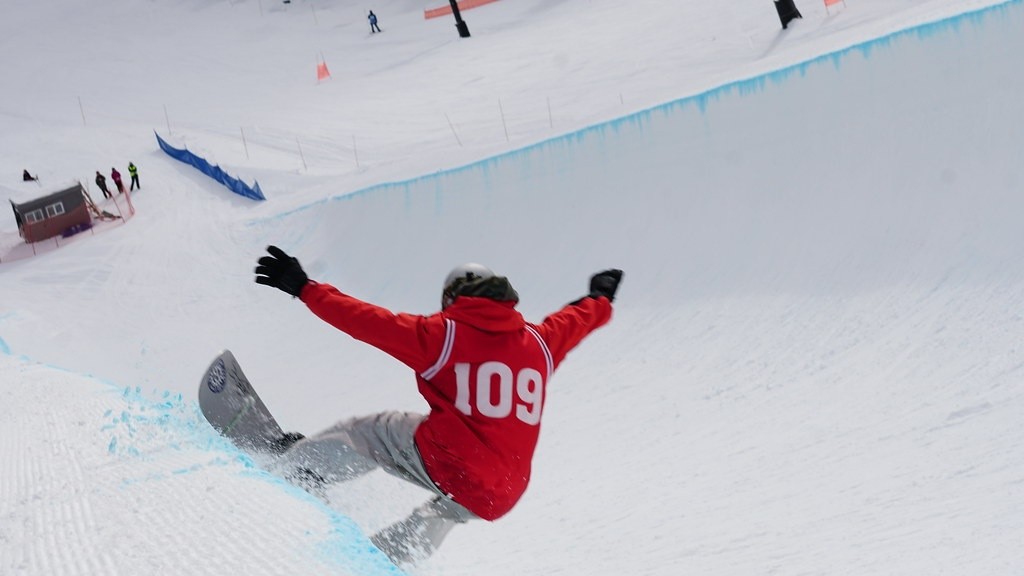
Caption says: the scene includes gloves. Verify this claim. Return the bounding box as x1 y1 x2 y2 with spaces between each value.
254 245 309 297
589 268 623 303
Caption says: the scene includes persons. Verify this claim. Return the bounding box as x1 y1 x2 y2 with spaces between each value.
255 246 623 568
96 171 112 199
128 162 140 191
368 11 381 33
112 168 123 194
24 170 34 181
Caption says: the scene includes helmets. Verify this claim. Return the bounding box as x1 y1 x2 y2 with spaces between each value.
441 261 495 292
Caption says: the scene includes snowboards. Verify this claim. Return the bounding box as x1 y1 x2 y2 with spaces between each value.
196 347 341 519
